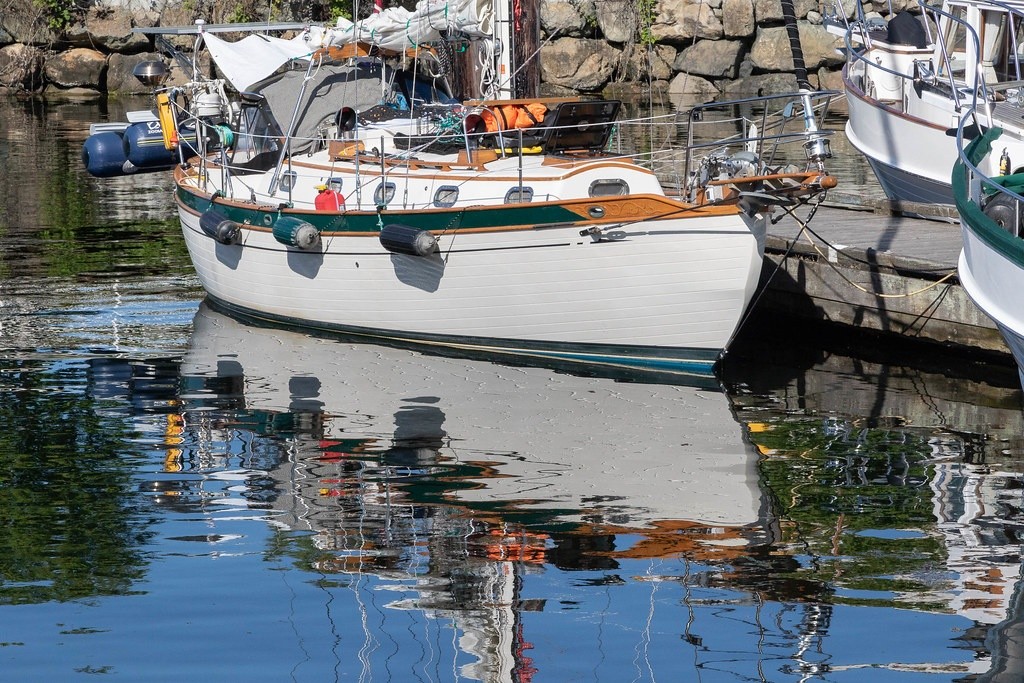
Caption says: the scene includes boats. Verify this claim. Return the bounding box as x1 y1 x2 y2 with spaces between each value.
81 0 848 374
799 0 1024 392
161 294 782 683
